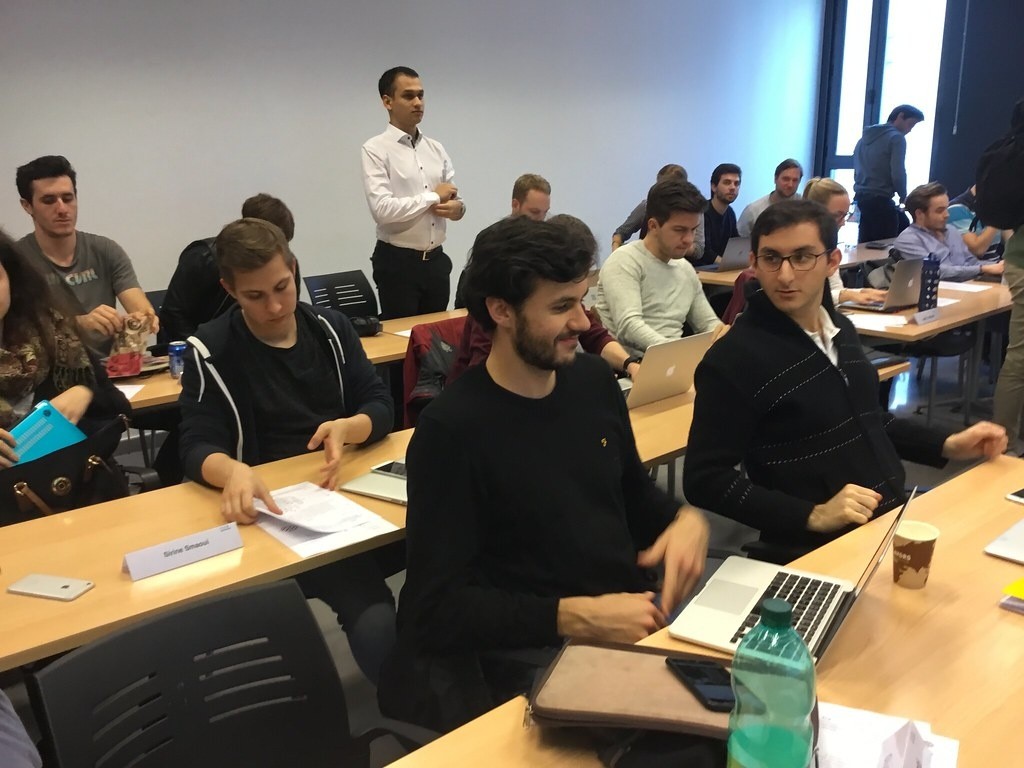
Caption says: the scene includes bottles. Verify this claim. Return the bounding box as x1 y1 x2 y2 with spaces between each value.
844 201 861 248
727 599 815 768
918 252 941 312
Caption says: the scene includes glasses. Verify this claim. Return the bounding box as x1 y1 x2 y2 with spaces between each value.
755 247 833 273
832 210 854 226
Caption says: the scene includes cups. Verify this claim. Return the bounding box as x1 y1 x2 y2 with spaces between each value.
893 520 939 589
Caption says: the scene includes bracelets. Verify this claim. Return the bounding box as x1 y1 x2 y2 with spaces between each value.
622 355 642 374
455 198 466 215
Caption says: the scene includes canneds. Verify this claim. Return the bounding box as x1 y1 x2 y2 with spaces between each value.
168 340 187 380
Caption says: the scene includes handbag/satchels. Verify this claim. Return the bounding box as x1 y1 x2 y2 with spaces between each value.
0 384 135 529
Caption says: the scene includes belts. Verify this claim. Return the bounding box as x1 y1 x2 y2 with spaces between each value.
376 240 443 261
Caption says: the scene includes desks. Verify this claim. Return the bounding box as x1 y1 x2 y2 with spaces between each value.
696 232 899 286
836 275 1014 426
626 361 912 501
0 425 417 673
111 331 409 468
382 453 1024 767
380 307 471 432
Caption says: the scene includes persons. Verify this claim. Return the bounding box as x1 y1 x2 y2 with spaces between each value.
371 66 465 321
142 195 301 494
455 159 850 308
976 98 1024 459
682 201 1010 566
892 180 998 280
406 174 728 425
400 213 711 698
177 216 394 688
853 105 925 245
0 229 133 527
13 156 160 359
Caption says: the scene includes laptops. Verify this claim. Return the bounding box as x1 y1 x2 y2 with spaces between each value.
616 330 715 411
839 257 925 311
695 236 752 272
667 485 918 670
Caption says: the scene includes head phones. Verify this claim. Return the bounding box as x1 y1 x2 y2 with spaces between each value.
349 315 384 337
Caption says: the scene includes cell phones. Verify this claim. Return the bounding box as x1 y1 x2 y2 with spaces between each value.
371 460 408 481
1005 489 1024 504
665 658 764 716
7 573 94 601
867 242 889 249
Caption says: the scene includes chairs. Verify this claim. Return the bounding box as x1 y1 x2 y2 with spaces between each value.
874 330 977 426
302 270 377 318
32 580 422 768
